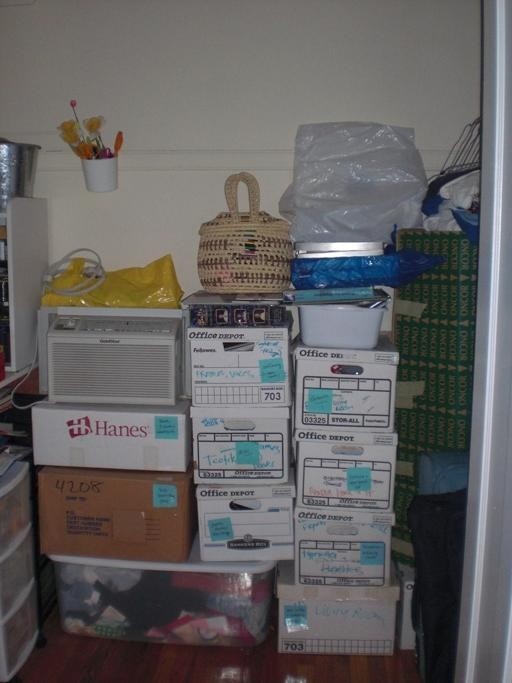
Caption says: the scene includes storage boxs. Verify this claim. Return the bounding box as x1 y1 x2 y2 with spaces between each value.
1 461 48 682
292 332 398 586
274 563 401 654
28 327 292 646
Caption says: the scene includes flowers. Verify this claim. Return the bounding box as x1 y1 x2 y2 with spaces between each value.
57 98 122 160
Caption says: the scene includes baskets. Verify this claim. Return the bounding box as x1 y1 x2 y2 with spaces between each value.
197 170 294 294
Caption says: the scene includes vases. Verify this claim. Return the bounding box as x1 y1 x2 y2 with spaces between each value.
79 159 118 194
